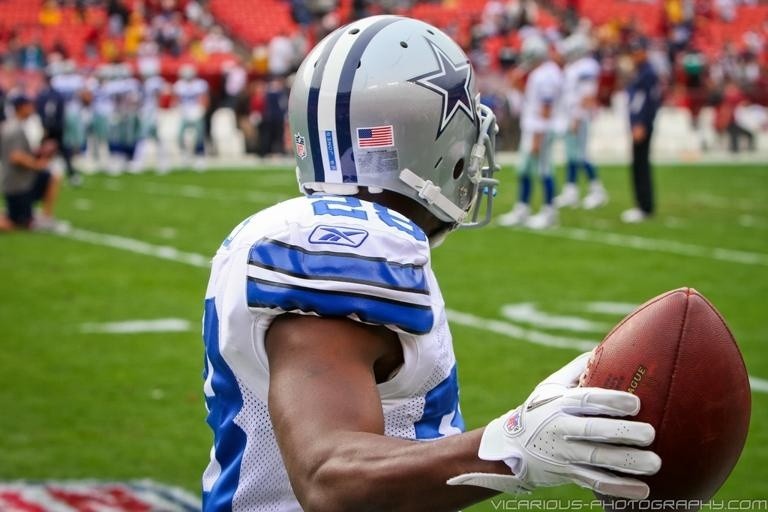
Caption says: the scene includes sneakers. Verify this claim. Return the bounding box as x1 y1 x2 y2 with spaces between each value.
552 182 580 208
620 207 649 223
524 204 559 231
581 178 610 209
496 201 532 228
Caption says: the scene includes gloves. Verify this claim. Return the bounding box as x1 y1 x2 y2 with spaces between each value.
445 351 662 501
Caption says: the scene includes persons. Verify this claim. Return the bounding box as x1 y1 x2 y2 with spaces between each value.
555 33 612 210
1 1 768 175
622 32 659 222
0 96 62 234
200 12 662 512
501 33 561 230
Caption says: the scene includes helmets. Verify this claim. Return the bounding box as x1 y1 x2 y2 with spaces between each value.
288 14 502 251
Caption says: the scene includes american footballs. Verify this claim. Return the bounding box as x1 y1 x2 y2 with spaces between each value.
581 287 752 512
